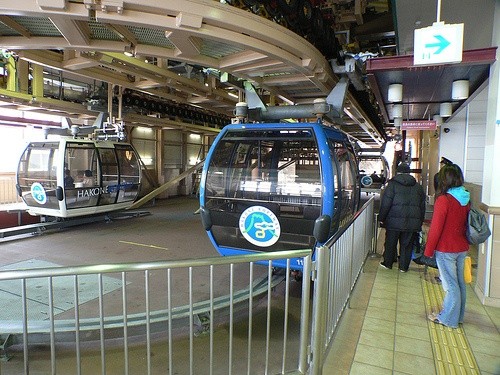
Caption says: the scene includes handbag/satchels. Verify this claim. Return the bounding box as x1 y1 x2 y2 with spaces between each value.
466 200 491 245
464 256 471 284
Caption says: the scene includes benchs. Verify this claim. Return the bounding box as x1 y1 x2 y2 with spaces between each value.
51 175 137 195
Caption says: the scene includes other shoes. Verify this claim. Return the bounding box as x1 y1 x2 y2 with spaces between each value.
379 262 389 269
428 314 442 323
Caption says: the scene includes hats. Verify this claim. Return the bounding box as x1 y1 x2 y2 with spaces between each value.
397 162 411 173
439 156 452 164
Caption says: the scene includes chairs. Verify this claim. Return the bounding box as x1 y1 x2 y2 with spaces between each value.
210 201 321 235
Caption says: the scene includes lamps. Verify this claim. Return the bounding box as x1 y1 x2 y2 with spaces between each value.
385 79 470 127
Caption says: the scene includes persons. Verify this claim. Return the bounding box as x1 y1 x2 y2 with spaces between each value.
74 170 101 196
433 157 453 192
423 164 471 328
378 163 426 274
64 169 74 187
368 169 387 183
